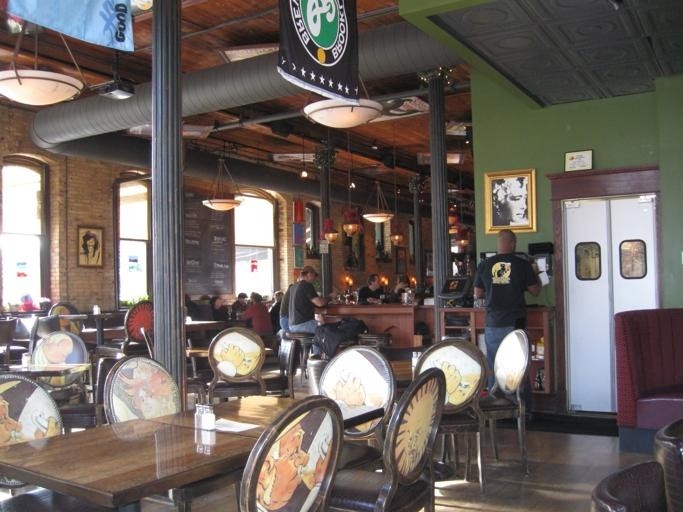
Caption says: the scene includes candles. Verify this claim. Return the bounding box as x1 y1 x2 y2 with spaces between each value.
348 278 353 300
344 276 349 295
381 276 388 289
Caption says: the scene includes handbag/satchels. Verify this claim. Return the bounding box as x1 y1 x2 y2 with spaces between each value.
336 318 369 340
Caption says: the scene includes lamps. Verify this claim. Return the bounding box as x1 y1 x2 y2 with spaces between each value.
93 50 137 101
0 20 88 106
300 116 308 177
202 132 242 211
303 71 383 128
320 129 409 246
291 177 307 223
454 197 471 248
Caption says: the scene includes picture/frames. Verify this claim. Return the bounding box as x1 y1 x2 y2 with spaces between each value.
76 224 105 268
484 167 538 236
292 224 305 269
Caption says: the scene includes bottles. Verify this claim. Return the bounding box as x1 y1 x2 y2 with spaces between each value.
93 304 100 315
22 353 32 368
193 404 216 430
194 429 216 456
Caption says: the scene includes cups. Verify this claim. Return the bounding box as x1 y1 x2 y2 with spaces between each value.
380 295 386 304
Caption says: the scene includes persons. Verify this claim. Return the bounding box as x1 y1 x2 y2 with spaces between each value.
472 229 541 414
280 276 302 331
186 292 284 342
358 274 384 304
289 266 328 335
494 177 527 225
19 295 39 311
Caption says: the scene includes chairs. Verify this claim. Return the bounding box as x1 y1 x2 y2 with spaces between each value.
590 416 683 511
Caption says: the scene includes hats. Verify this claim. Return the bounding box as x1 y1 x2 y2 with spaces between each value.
301 266 319 277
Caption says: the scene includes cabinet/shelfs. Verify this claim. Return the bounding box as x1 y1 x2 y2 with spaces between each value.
438 306 558 396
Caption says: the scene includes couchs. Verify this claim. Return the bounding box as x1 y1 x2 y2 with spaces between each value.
614 307 682 455
